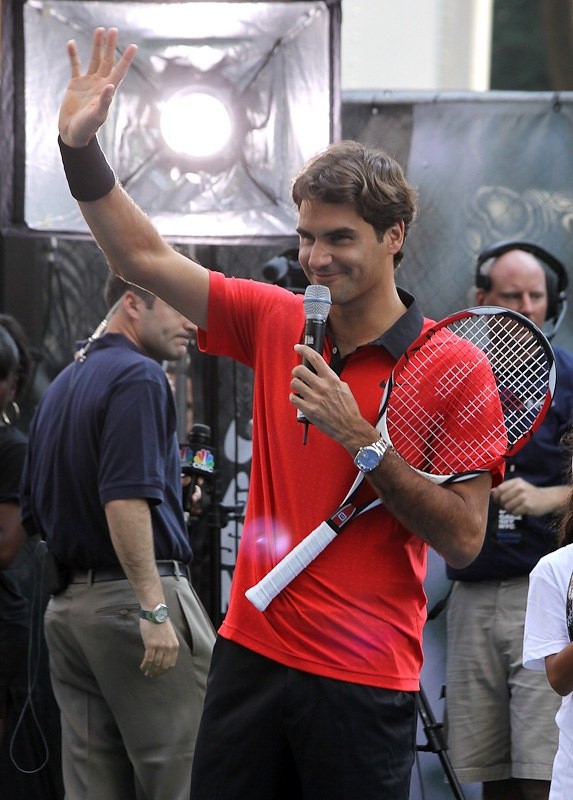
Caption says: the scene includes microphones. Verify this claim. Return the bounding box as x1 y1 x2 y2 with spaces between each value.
543 292 568 343
295 284 332 447
179 422 217 523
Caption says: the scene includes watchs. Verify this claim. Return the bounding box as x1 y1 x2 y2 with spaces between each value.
354 436 393 473
141 603 168 624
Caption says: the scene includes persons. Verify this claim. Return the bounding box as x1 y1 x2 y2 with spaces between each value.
446 242 573 800
56 27 506 800
0 313 64 800
19 242 218 800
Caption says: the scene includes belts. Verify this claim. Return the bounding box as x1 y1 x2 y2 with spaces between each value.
70 562 188 585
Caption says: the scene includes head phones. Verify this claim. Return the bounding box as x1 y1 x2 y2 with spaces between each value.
474 240 569 326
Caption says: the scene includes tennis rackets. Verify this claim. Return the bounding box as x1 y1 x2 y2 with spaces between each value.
242 305 559 613
